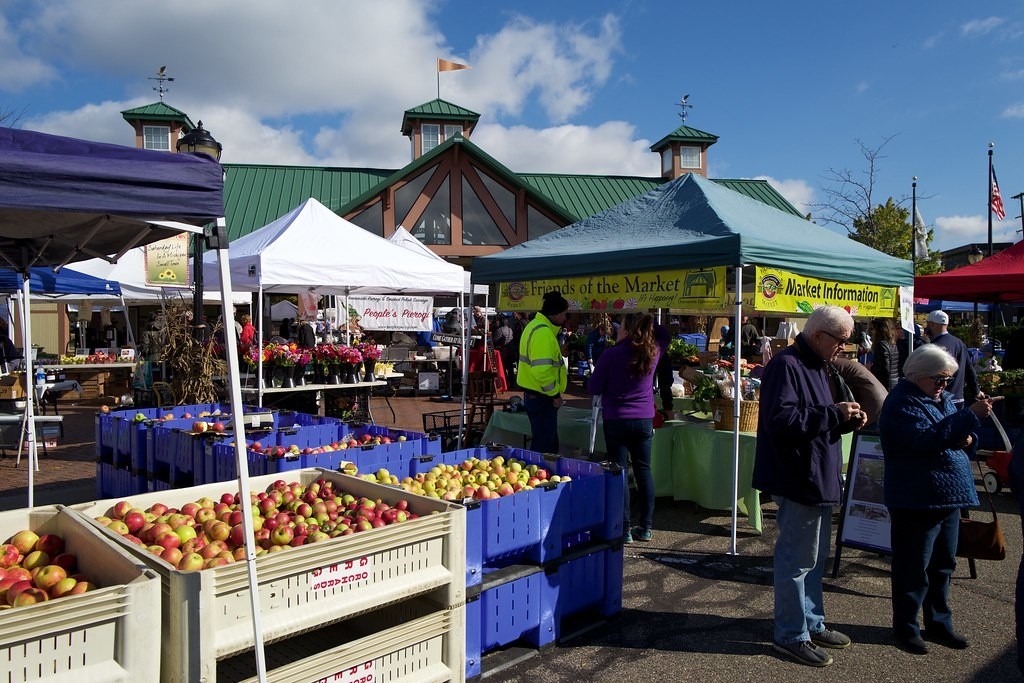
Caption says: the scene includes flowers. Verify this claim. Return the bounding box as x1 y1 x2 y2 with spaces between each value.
243 341 382 371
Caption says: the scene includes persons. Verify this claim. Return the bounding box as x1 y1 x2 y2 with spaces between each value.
581 312 664 542
718 315 761 361
652 320 675 418
751 303 867 668
186 304 444 384
878 343 1005 653
516 290 569 454
472 306 536 389
584 322 611 368
830 308 986 431
982 343 1003 372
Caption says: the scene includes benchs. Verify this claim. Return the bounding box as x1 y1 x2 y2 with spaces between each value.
0 412 64 468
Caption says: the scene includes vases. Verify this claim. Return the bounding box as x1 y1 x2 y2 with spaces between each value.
253 358 378 388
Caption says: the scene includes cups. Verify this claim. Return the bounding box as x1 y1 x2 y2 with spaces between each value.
409 351 418 360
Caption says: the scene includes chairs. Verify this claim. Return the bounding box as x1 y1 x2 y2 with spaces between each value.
462 371 512 448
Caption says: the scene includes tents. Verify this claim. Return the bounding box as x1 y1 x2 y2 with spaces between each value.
0 126 268 683
158 197 470 447
914 297 995 324
11 245 253 325
0 266 148 391
329 225 490 371
468 171 917 553
913 237 1024 359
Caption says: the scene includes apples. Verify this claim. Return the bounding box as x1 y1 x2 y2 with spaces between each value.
130 410 408 457
94 480 442 571
343 456 573 501
60 351 134 365
0 530 102 611
100 405 109 413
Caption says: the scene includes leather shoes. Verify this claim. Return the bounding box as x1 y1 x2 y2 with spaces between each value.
890 629 932 655
925 629 970 647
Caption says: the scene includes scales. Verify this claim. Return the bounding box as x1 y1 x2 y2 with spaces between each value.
225 410 275 430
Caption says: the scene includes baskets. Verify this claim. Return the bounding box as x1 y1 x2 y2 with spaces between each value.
708 397 759 431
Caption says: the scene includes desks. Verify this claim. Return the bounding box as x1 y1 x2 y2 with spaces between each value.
34 380 84 456
33 363 138 368
225 380 387 425
480 406 853 536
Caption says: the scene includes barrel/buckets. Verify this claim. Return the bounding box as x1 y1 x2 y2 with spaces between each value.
700 351 718 366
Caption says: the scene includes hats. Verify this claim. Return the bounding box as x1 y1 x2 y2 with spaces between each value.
541 291 569 315
926 309 949 324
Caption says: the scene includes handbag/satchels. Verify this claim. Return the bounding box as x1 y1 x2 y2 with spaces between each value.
955 518 1006 560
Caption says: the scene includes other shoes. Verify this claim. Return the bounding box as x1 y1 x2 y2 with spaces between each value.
631 525 652 540
623 530 633 543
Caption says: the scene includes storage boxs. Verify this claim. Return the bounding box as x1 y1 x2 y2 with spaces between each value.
432 346 458 359
383 347 408 360
375 372 404 389
0 373 28 398
678 333 707 352
770 338 794 355
0 401 628 683
62 371 112 400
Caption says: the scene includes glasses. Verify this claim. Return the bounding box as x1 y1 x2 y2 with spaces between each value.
930 376 954 386
821 330 849 345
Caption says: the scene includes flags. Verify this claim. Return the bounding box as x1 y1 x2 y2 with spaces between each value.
914 207 928 258
991 165 1005 218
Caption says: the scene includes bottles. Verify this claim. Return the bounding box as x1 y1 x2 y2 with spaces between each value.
741 377 756 401
66 337 76 357
14 363 65 385
76 342 81 354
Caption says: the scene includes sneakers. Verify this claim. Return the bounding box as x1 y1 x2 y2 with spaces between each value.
811 626 851 648
772 640 833 666
